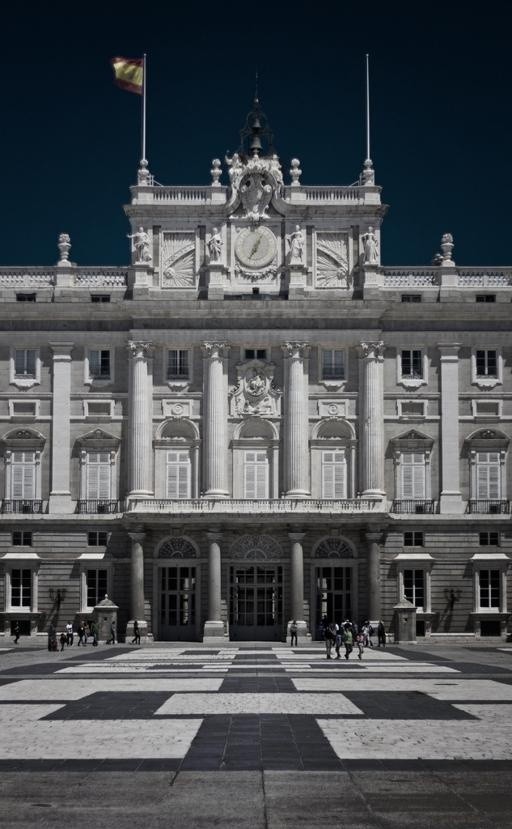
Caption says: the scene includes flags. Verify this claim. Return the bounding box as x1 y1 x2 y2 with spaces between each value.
111 55 144 96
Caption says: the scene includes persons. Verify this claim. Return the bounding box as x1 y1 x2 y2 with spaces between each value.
317 614 387 660
207 228 224 261
283 224 306 263
13 620 21 644
126 226 152 262
289 618 299 647
46 616 142 652
359 226 379 263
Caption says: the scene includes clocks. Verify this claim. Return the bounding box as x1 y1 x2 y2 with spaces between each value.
233 226 278 267
